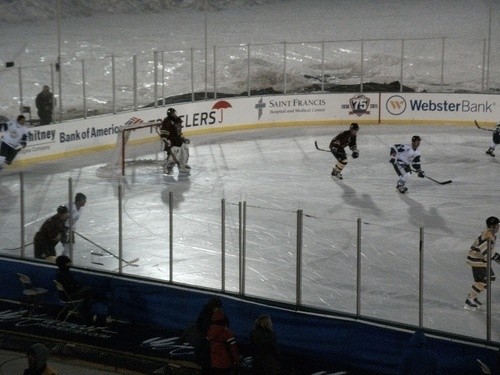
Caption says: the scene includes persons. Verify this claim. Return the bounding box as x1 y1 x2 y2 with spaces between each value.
485 123 500 158
33 204 69 260
160 108 191 176
54 256 92 317
389 136 424 194
0 115 30 173
464 217 500 311
35 84 57 126
249 314 281 375
61 192 86 257
23 344 58 375
208 309 240 375
397 329 442 375
329 123 359 179
197 297 230 371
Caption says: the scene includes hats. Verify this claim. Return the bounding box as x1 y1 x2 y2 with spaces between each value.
486 216 500 228
75 193 86 202
412 135 421 142
350 123 359 130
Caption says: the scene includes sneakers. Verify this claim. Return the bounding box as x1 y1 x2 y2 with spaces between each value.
474 298 482 306
331 169 342 180
396 185 405 194
463 299 478 311
401 185 408 191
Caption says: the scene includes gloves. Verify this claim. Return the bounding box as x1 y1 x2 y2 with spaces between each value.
418 171 425 178
389 158 396 164
352 152 359 158
332 147 337 153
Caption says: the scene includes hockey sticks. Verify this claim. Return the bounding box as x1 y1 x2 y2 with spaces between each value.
394 162 452 185
314 140 356 156
474 119 499 132
71 228 140 265
157 131 192 172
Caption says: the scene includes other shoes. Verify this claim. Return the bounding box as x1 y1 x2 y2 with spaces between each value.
486 151 493 155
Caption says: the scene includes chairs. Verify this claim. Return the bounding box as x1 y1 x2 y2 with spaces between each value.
476 359 491 375
20 107 32 125
17 273 50 318
52 280 86 323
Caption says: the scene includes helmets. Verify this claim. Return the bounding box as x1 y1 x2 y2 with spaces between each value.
167 108 176 117
57 205 69 214
